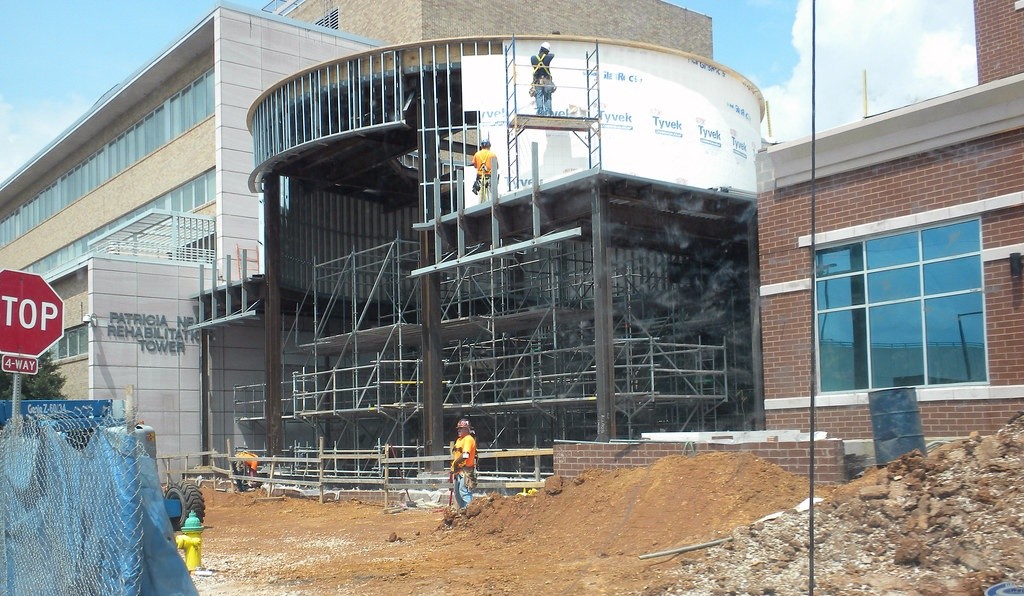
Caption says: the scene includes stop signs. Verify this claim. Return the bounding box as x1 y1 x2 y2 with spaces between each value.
0 268 64 358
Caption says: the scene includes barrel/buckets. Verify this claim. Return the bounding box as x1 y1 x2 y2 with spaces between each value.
869 387 926 465
984 582 1024 596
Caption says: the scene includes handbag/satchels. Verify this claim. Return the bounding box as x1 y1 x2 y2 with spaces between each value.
540 78 557 94
529 82 538 97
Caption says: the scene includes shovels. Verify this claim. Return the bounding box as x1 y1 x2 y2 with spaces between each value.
390 444 417 508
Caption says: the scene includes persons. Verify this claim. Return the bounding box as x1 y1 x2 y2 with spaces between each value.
231 452 267 493
471 140 499 203
531 42 555 116
450 418 477 509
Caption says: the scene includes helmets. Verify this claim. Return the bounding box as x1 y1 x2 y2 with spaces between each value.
456 418 471 429
481 139 491 147
541 41 550 52
